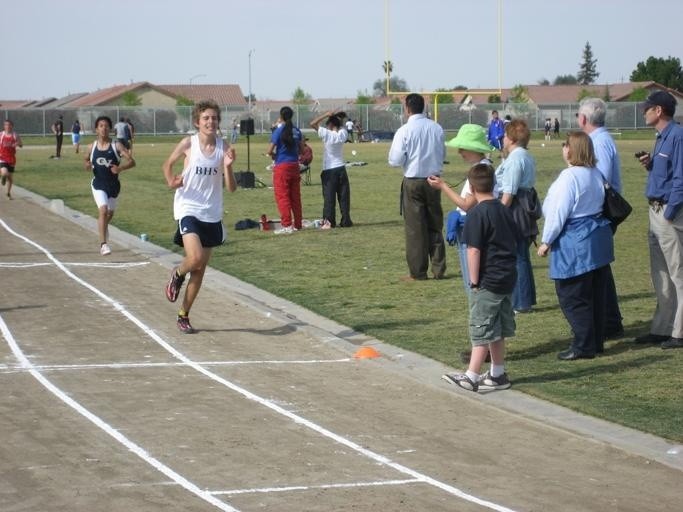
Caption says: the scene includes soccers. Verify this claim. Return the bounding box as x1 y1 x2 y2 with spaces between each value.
352 150 356 155
151 143 154 146
541 143 545 147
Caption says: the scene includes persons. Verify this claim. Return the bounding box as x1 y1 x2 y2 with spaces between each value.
493 120 537 314
71 120 81 153
84 115 136 256
635 91 683 349
162 99 238 334
345 118 356 143
537 131 614 361
427 122 498 364
387 93 446 279
503 114 512 131
575 94 625 345
309 111 353 228
113 117 134 159
266 105 303 234
545 118 552 141
554 118 560 137
124 118 134 159
51 114 64 157
352 119 364 142
439 163 520 396
231 117 237 144
298 134 313 171
487 110 505 163
0 120 22 200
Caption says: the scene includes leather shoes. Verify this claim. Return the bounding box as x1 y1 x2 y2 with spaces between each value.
608 324 625 340
558 346 597 361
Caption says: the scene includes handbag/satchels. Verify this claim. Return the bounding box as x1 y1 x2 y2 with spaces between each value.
602 179 632 225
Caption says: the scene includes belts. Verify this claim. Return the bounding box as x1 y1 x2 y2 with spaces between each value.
648 199 665 205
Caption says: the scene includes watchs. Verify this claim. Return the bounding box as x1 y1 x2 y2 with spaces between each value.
468 280 481 288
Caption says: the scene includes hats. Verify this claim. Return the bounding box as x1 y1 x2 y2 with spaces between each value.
302 135 309 141
445 123 495 154
636 90 677 112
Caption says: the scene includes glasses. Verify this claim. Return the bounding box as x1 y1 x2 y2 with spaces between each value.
562 143 570 148
576 113 581 118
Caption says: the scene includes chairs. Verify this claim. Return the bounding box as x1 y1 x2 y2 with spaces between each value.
300 161 312 184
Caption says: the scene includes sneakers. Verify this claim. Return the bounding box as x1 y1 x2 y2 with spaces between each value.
434 273 443 279
660 338 683 349
176 313 193 334
2 177 6 186
442 374 479 394
100 243 111 256
461 351 491 364
293 227 301 231
166 266 186 303
478 371 511 390
274 227 294 235
400 276 417 282
7 193 11 200
635 333 670 343
